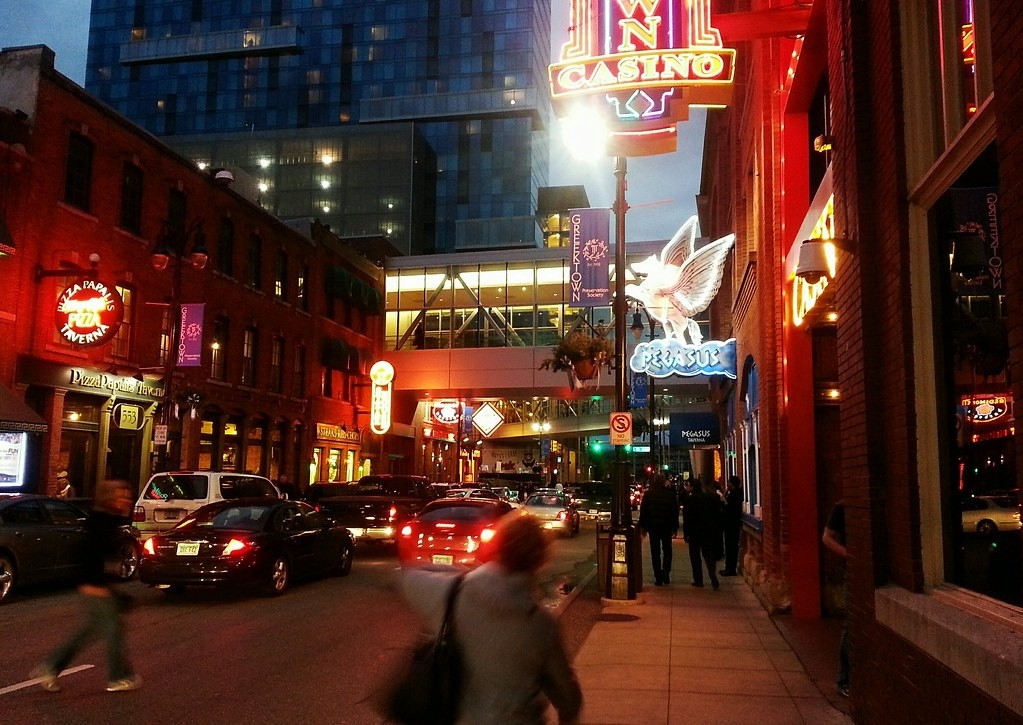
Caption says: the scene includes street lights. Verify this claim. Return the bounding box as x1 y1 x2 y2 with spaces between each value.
568 109 645 606
533 421 550 463
630 300 656 479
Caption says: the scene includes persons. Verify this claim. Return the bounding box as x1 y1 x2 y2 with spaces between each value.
666 477 727 588
518 481 525 502
555 481 563 494
597 319 605 330
823 499 855 697
33 478 143 692
527 480 534 496
637 474 680 585
56 468 77 498
719 476 744 575
401 516 584 725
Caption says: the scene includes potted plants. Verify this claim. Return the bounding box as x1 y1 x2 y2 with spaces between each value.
538 333 617 380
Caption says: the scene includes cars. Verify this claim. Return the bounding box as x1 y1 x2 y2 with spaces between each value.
962 496 1023 537
0 494 142 603
303 473 644 571
139 497 354 597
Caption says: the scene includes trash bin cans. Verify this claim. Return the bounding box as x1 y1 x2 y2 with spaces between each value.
597 522 643 593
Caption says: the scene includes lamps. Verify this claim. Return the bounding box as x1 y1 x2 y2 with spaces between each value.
35 252 100 284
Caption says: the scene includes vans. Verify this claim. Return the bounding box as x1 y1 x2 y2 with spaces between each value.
132 470 288 546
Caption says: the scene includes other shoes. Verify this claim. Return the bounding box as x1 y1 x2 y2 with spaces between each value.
841 687 849 696
655 581 662 586
711 580 719 590
106 676 142 692
691 581 704 588
661 570 670 585
29 664 62 692
719 568 736 576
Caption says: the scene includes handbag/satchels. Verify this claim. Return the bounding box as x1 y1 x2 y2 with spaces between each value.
381 573 465 725
